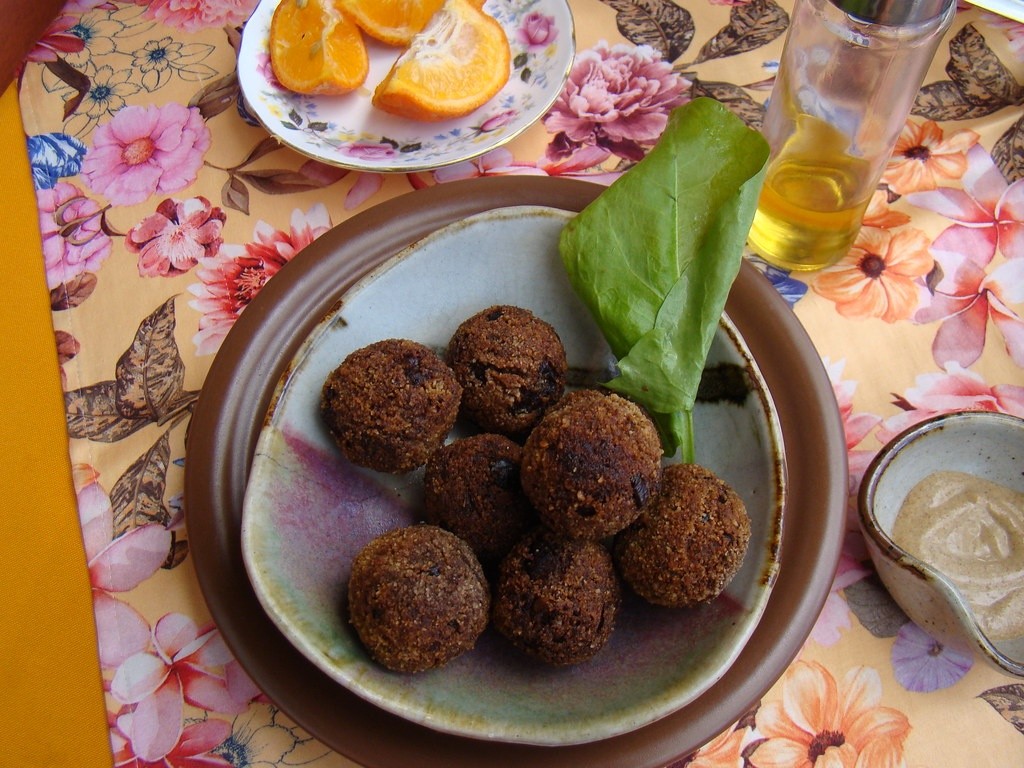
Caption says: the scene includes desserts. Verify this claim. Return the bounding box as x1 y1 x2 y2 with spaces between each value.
324 305 750 673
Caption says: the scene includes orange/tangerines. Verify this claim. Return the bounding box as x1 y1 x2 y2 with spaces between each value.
270 0 510 122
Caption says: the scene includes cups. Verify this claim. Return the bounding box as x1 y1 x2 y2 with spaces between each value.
748 0 962 277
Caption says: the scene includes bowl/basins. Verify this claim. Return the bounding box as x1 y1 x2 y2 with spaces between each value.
856 410 1024 680
183 175 852 768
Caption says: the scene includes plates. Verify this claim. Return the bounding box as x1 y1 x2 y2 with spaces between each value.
236 1 580 177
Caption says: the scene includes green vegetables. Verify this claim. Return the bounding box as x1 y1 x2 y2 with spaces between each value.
559 97 771 465
